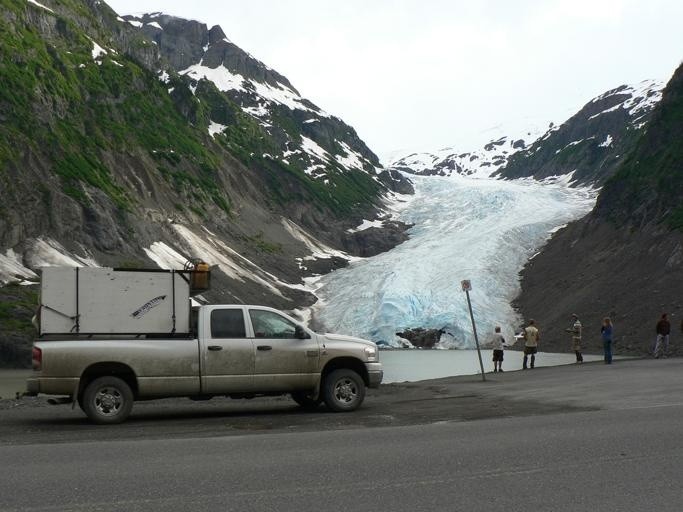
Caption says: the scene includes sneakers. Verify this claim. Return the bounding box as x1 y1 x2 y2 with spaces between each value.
494 368 502 372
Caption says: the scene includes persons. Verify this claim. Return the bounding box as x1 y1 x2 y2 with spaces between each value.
522 319 540 369
654 313 670 359
599 317 613 363
565 314 583 363
491 327 505 372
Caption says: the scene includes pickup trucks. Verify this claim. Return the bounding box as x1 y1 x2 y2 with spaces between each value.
25 304 384 423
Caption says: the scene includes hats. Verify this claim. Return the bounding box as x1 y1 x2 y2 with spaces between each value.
528 319 538 324
603 317 610 321
662 314 666 319
571 313 579 318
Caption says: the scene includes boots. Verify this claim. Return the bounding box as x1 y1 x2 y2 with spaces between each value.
529 356 535 368
522 356 527 369
